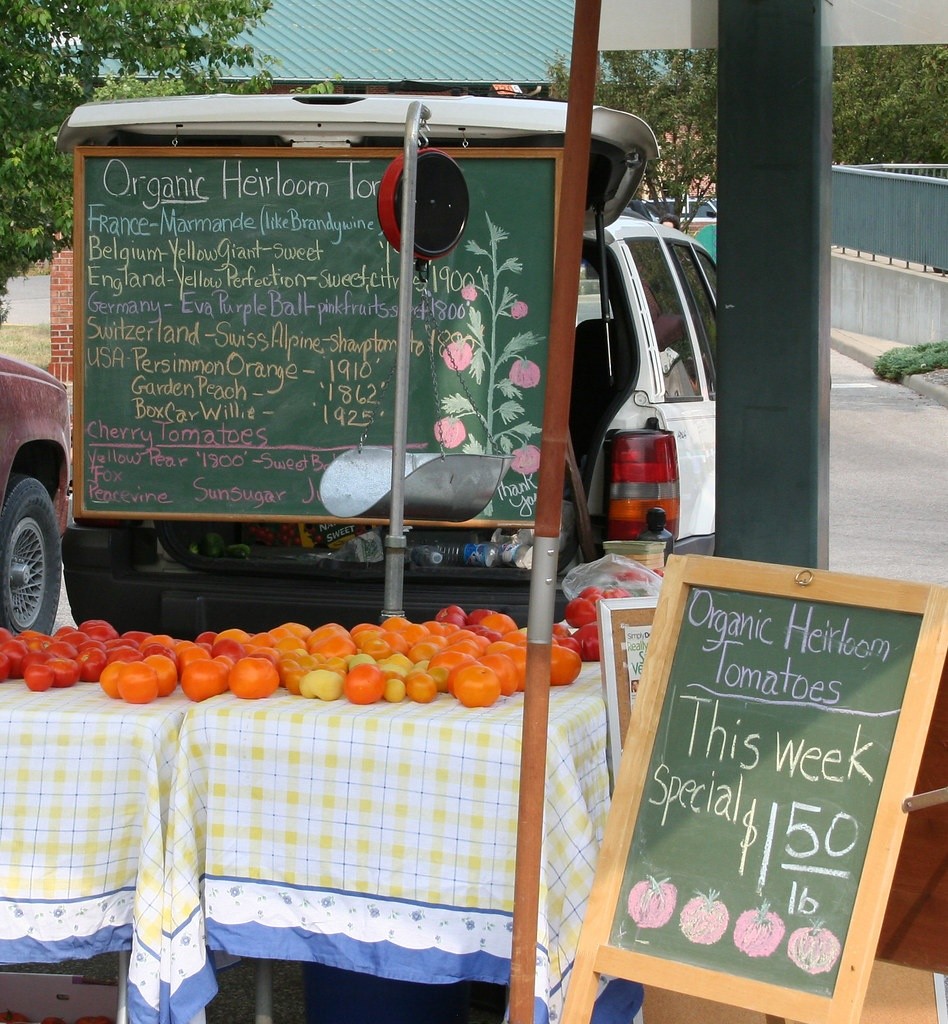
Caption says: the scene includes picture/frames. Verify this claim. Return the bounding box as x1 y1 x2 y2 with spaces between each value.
70 143 565 530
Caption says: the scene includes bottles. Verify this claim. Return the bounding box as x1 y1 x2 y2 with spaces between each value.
404 545 442 568
438 543 499 568
639 507 674 567
499 543 530 568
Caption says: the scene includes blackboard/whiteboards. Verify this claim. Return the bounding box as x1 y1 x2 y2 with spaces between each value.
582 551 948 1024
71 145 565 527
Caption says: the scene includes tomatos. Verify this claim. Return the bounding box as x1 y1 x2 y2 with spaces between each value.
0 569 665 710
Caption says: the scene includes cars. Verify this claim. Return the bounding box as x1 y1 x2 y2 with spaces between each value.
0 356 73 637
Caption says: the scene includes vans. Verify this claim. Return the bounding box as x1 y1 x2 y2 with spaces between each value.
639 198 717 224
66 87 720 645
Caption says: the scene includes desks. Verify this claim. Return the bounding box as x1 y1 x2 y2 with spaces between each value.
0 663 647 1024
